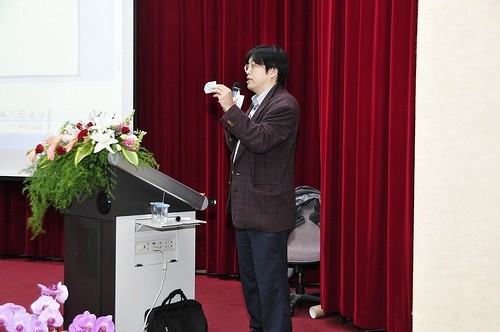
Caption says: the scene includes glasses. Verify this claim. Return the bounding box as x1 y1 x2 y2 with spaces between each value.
243 63 261 72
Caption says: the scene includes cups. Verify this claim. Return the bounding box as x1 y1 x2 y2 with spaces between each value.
155 203 170 224
150 203 157 222
309 305 325 319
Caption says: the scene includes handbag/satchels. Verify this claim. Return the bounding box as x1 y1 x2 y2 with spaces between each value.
144 289 208 332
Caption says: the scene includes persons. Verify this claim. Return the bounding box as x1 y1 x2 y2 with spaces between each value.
211 45 300 332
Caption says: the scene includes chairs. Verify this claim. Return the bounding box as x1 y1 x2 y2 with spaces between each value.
286 186 321 315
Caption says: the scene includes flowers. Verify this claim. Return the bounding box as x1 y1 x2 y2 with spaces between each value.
0 282 115 332
21 113 159 242
